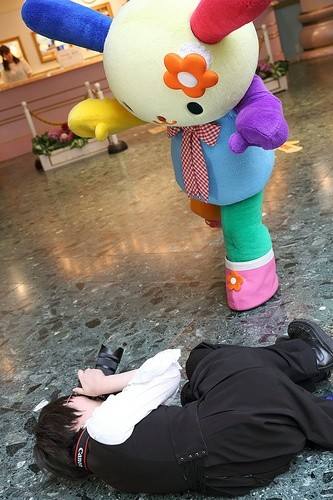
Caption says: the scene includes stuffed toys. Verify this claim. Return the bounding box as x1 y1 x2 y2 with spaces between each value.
21 0 289 311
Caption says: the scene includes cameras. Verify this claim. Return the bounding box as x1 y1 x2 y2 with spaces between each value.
76 344 124 400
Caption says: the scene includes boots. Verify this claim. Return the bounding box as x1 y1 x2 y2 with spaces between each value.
287 318 333 373
311 370 331 384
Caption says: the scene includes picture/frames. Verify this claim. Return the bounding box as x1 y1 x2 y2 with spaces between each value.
31 2 114 63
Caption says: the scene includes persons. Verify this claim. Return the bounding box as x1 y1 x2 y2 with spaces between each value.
0 45 32 83
33 318 333 497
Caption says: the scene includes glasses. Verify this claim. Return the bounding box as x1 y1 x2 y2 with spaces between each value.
64 392 78 406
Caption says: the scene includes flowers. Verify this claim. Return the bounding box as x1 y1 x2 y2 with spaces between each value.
31 123 88 157
255 55 288 80
